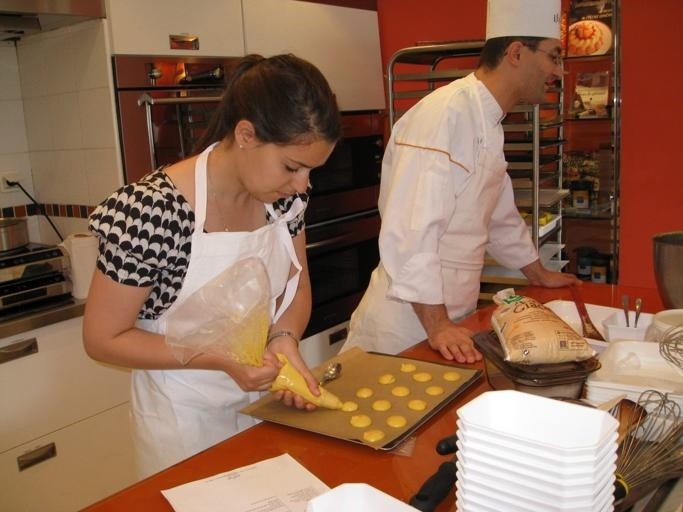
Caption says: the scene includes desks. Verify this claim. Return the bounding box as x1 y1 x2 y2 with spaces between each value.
80 281 683 512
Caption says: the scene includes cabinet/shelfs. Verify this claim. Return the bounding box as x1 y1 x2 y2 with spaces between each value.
387 39 566 310
101 0 245 59
0 297 146 511
562 0 621 283
242 0 387 116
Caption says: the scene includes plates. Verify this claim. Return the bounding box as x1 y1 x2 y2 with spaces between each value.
564 19 613 58
453 388 626 512
304 479 425 511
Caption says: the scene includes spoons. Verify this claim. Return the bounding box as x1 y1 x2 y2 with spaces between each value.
318 362 342 389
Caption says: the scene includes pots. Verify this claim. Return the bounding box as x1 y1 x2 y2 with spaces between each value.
1 216 34 253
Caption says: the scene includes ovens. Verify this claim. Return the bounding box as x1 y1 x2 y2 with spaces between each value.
273 110 391 339
110 48 275 194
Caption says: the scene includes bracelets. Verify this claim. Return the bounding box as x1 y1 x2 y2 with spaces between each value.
266 331 299 347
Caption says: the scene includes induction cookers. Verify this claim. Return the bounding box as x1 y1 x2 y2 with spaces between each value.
0 241 66 261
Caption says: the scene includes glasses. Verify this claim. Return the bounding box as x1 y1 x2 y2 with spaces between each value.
504 43 563 67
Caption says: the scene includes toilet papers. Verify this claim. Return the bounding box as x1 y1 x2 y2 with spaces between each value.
61 231 96 301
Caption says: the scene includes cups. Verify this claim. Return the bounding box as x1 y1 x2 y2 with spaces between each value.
569 181 592 210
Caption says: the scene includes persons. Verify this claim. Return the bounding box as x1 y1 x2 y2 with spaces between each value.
83 53 341 482
337 0 583 363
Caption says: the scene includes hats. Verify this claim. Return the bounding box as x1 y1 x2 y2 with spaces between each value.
484 0 563 45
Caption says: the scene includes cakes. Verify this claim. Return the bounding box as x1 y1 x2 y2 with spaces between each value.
568 21 605 54
